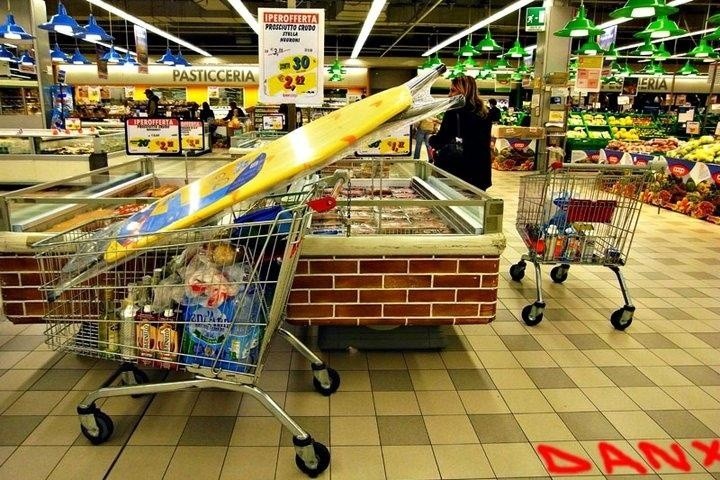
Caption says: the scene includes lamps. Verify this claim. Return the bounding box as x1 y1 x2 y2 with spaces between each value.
472 3 505 51
651 38 672 61
326 15 347 83
553 1 606 37
0 0 193 67
417 35 537 84
703 26 720 41
459 12 482 57
634 32 656 56
574 2 609 54
604 7 621 56
686 35 719 63
503 0 531 59
641 15 687 37
609 1 680 19
569 57 699 84
708 15 719 24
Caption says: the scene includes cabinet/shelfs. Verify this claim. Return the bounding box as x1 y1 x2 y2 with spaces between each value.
491 106 720 223
1 69 340 140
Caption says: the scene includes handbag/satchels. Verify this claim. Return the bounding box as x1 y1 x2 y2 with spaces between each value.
432 110 464 160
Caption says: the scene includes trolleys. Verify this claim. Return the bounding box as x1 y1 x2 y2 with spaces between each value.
31 169 351 477
509 161 650 329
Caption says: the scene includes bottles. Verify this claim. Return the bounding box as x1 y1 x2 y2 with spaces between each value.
229 287 261 368
560 224 577 263
542 225 559 262
98 288 120 358
120 268 179 371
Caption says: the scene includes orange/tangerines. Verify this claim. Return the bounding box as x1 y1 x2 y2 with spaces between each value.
609 115 637 139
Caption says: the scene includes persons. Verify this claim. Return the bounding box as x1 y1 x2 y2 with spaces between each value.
412 114 434 163
200 101 217 134
144 88 159 118
488 98 502 122
223 101 246 147
183 103 200 121
427 74 491 193
